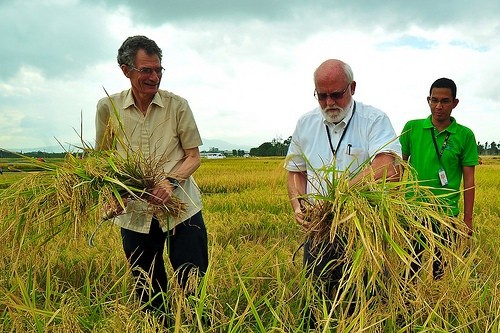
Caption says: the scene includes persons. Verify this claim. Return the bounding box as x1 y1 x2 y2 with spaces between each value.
283 59 403 329
389 78 480 280
95 35 214 332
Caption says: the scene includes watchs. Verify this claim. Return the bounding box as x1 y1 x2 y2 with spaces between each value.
166 177 179 190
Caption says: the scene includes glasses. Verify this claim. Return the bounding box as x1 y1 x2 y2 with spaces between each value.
133 67 165 74
428 97 454 105
314 82 350 101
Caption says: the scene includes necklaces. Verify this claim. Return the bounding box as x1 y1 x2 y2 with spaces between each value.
325 99 356 154
